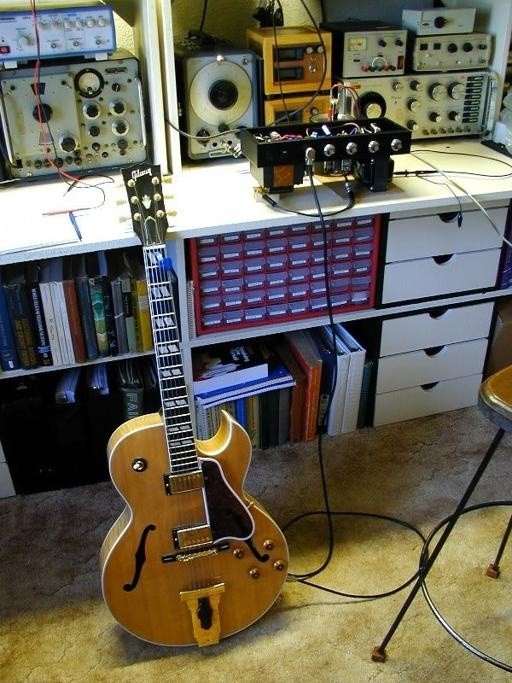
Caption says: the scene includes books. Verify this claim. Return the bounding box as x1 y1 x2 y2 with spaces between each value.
0 248 183 373
192 321 374 452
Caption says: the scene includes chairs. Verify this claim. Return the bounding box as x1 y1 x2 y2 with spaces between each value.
371 368 511 674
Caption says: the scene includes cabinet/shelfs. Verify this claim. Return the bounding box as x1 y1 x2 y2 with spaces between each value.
155 0 512 450
2 0 174 499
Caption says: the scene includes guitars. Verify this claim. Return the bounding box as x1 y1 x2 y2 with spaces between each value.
100 166 289 649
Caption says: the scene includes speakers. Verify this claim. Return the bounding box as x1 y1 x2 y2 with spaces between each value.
175 36 257 164
